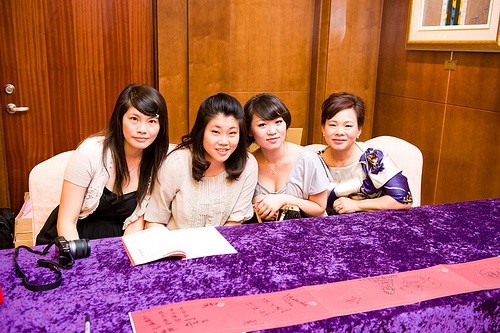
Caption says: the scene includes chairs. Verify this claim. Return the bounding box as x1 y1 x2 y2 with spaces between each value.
366 136 423 206
28 151 73 246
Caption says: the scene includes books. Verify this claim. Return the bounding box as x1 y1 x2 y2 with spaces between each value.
121 225 238 267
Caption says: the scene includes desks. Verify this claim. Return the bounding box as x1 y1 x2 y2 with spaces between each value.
0 197 500 333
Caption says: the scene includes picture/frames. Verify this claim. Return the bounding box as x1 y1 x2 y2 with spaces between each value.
405 0 500 52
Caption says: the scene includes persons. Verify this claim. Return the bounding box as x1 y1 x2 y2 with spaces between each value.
316 92 413 216
241 94 329 223
35 83 170 246
143 93 258 230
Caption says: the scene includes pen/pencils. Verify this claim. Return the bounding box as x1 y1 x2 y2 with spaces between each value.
84 314 90 333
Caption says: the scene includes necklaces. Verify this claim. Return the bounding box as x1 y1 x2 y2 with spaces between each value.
332 145 356 174
263 143 286 174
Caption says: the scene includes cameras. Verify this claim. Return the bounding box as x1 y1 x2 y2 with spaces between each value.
55 236 91 267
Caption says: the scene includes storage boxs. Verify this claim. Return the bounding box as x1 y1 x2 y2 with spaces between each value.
13 191 33 248
